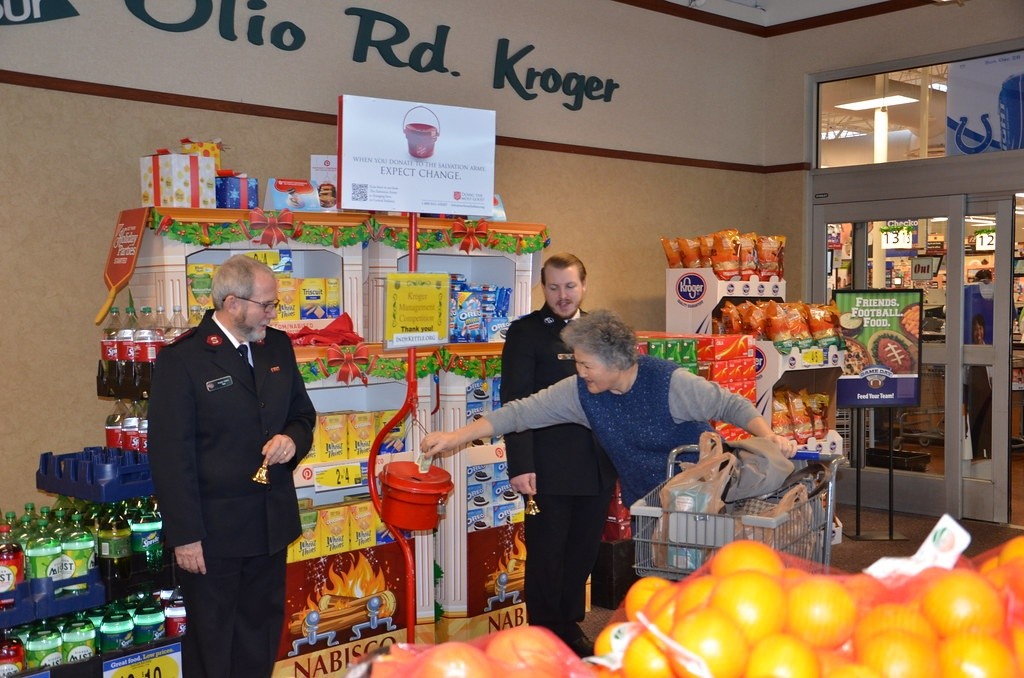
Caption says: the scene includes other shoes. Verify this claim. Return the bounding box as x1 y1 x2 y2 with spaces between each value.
546 622 596 658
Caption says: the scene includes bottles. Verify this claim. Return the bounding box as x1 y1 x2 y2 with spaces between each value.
0 496 186 678
101 305 208 467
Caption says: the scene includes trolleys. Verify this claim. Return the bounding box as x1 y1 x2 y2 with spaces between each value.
629 444 846 581
892 367 944 451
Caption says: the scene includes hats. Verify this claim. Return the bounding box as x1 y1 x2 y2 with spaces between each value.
971 270 993 283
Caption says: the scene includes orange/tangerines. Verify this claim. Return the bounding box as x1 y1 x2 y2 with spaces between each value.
422 627 574 678
591 538 1024 678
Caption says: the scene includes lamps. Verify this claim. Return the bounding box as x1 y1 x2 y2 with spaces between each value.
833 90 920 112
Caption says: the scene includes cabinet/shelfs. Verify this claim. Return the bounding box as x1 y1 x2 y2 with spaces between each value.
665 267 844 548
118 206 549 658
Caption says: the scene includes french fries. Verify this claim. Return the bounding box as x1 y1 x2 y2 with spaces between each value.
902 306 919 339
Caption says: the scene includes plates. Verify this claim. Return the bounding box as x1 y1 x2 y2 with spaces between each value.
867 332 913 367
900 303 920 342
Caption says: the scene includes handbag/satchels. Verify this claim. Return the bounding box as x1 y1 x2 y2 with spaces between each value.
732 485 818 563
649 430 737 570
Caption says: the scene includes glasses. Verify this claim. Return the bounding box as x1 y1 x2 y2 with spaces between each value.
222 296 281 313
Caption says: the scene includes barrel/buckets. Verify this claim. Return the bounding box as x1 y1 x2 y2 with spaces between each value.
378 419 456 531
402 105 441 159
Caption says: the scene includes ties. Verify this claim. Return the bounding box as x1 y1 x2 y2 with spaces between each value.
239 344 249 367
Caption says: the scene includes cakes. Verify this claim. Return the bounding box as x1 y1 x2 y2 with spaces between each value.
871 332 916 374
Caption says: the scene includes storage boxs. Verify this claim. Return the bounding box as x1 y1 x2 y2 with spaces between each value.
847 447 932 473
36 447 155 502
29 566 106 620
0 582 35 629
139 140 525 563
96 358 155 400
96 551 176 602
602 331 758 543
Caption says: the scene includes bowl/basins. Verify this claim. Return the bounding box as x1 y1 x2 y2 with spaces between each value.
839 312 864 336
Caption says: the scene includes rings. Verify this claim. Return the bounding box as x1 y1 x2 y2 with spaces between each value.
283 450 288 455
787 442 792 446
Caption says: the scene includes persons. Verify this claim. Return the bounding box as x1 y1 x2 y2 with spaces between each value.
146 254 316 678
963 315 993 460
956 268 1018 396
418 309 798 544
500 253 619 658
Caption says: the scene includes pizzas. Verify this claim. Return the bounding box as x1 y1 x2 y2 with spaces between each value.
841 336 872 376
